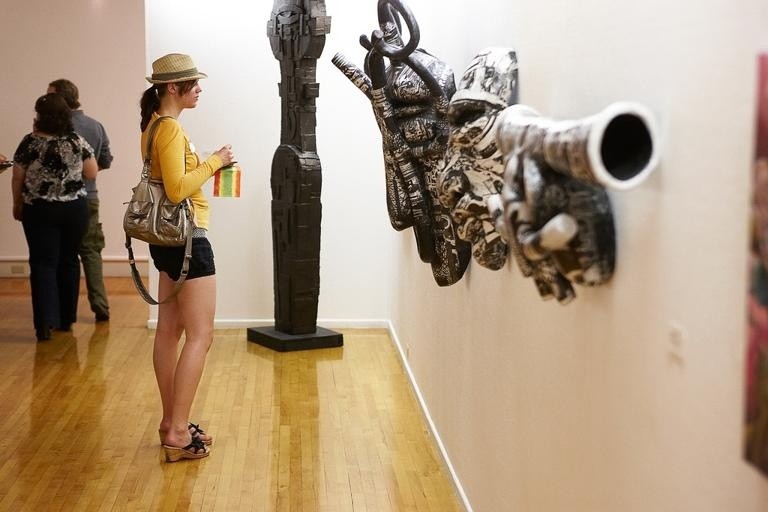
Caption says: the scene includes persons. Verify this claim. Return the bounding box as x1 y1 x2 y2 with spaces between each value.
46 79 115 324
11 92 101 342
140 52 235 462
0 153 13 175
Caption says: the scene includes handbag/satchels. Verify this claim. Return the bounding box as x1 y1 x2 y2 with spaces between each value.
120 114 197 249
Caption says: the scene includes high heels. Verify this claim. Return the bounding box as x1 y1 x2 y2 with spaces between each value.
162 440 211 463
156 421 212 446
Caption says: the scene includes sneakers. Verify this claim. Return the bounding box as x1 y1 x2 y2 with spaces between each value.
35 326 52 341
90 301 111 321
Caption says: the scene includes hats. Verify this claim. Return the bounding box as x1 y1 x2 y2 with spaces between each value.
144 53 208 85
